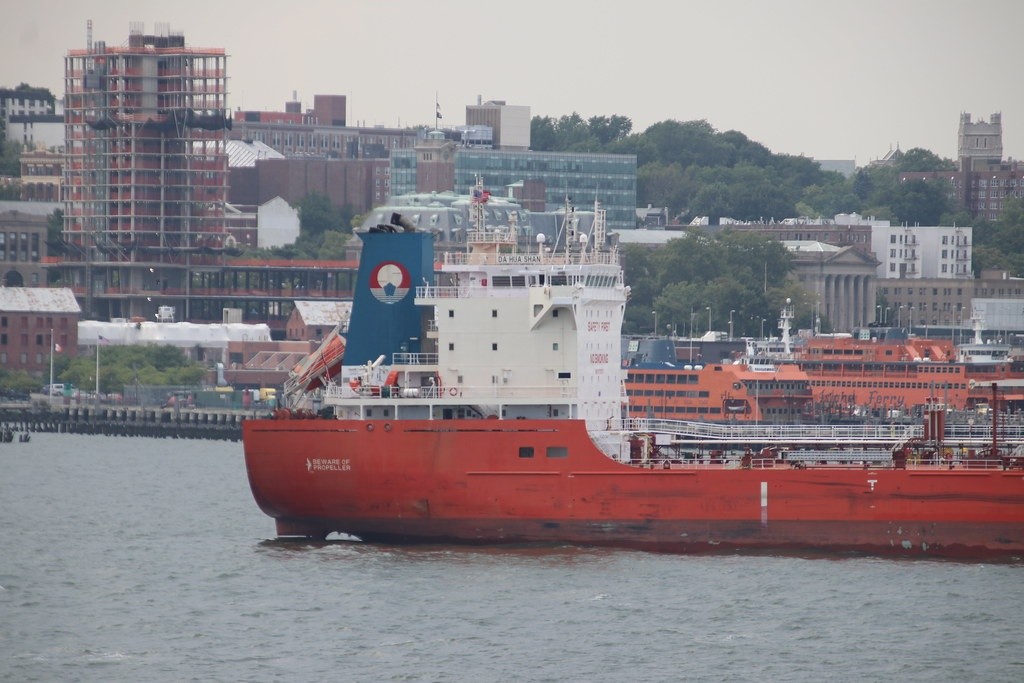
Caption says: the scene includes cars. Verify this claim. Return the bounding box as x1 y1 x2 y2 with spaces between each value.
41 385 50 394
53 384 78 396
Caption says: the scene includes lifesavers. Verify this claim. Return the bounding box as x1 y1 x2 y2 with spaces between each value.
449 388 458 396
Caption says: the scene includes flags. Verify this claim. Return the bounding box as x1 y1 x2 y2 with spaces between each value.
98 335 113 346
436 103 442 118
54 343 62 353
471 190 490 203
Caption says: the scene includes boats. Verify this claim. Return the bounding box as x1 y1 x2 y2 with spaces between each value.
285 330 349 391
244 170 1023 558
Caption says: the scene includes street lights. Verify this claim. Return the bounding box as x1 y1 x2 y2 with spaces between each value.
730 310 735 341
951 305 957 347
960 306 967 345
884 307 892 325
898 305 904 327
705 306 711 332
761 318 767 342
910 306 915 332
877 305 882 325
651 311 658 339
